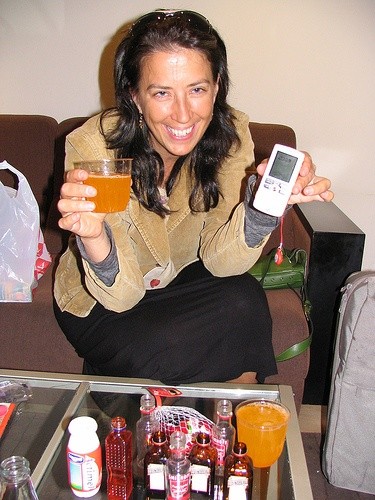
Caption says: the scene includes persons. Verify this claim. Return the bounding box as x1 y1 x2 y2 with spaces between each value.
54 11 334 416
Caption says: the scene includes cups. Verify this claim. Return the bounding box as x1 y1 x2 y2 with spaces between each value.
80 158 133 213
234 399 290 468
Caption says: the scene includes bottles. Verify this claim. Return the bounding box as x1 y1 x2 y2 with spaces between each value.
189 432 218 494
0 456 39 500
165 431 192 500
224 443 253 500
210 400 236 478
144 431 167 500
135 394 159 469
66 416 102 498
104 417 133 500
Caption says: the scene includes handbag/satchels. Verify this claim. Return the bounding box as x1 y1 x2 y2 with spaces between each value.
0 159 52 303
246 247 308 291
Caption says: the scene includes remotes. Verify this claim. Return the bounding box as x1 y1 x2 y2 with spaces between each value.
253 144 305 217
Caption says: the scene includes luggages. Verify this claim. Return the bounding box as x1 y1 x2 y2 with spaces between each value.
320 270 374 495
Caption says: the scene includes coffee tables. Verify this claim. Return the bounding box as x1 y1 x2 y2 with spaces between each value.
0 369 315 500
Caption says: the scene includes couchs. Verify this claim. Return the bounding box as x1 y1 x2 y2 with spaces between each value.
0 115 366 416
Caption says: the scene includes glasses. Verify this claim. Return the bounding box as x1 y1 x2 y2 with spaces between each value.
130 11 212 40
